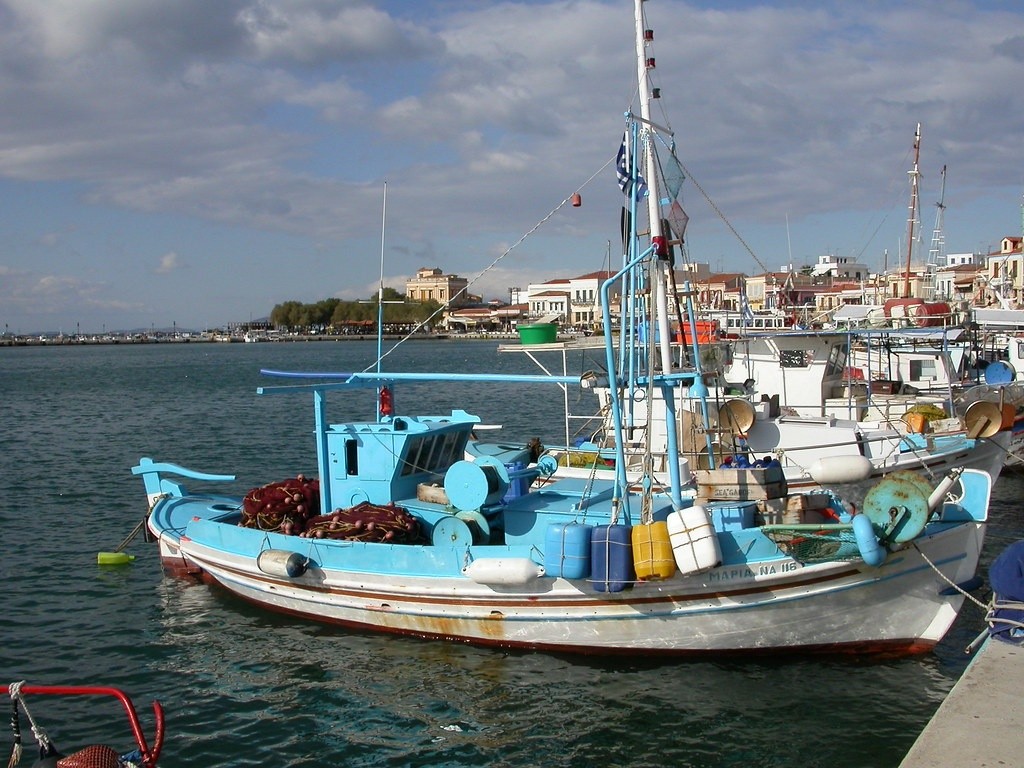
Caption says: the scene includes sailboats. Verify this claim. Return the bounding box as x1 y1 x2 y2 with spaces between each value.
131 0 993 664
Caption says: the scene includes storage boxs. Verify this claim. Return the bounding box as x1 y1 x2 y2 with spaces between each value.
677 318 720 345
691 466 831 532
870 380 894 395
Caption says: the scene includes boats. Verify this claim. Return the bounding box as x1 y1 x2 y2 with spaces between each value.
583 116 1024 502
